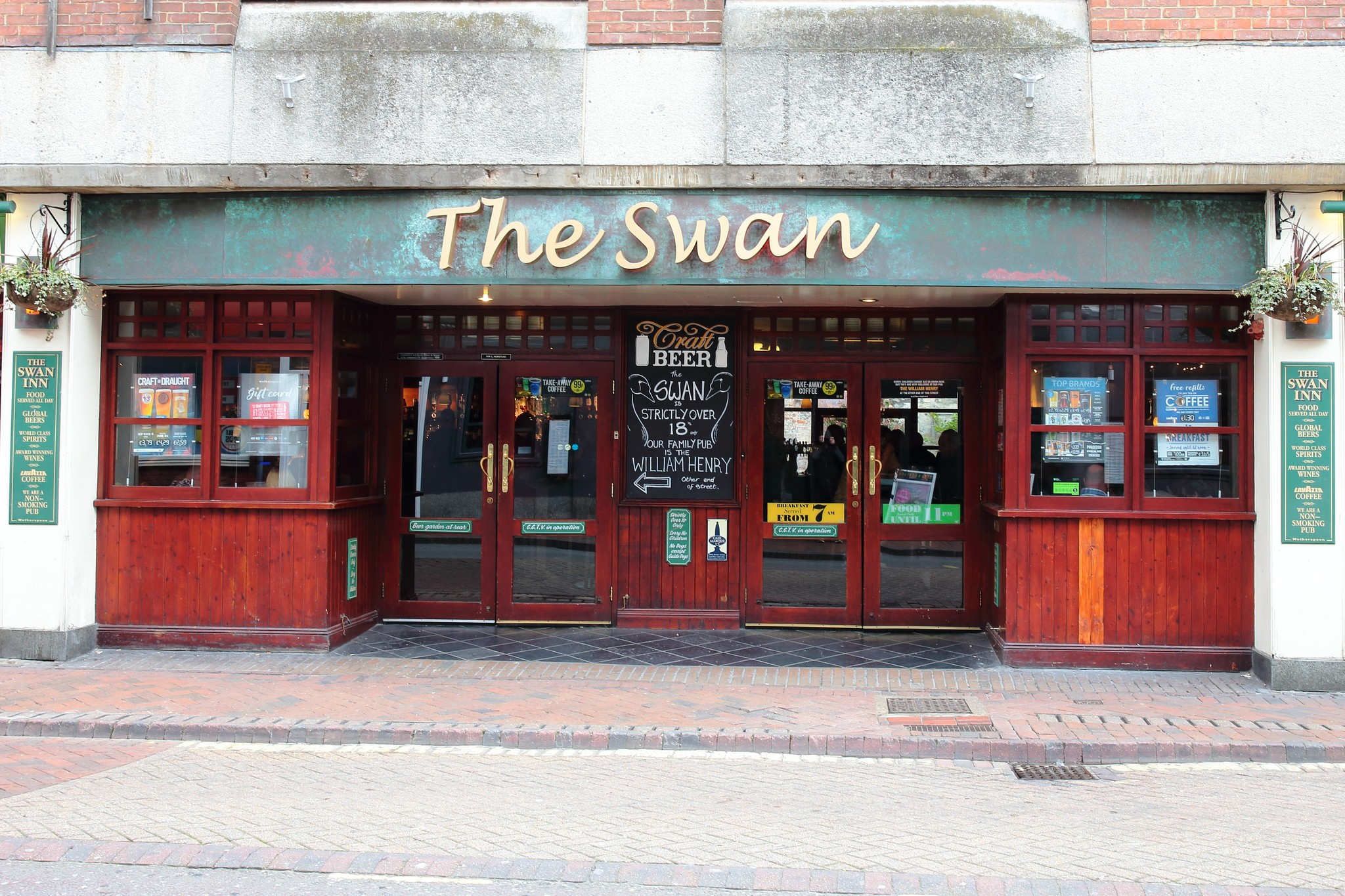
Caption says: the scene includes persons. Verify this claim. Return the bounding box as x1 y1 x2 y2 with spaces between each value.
808 425 847 522
265 448 297 487
515 412 538 453
1144 465 1175 497
187 464 200 487
880 425 963 522
1080 464 1109 496
428 408 470 455
1178 476 1212 498
763 431 797 522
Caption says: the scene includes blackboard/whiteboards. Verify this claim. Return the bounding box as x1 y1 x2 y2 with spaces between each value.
619 305 742 508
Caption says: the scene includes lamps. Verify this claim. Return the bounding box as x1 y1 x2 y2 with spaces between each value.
439 393 452 409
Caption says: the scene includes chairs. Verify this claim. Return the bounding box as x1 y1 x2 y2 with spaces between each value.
403 406 418 441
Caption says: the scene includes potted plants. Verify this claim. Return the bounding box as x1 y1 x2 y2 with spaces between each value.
0 211 107 343
1228 210 1345 332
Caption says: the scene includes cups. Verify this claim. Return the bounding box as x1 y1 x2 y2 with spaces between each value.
155 389 171 428
138 388 155 427
767 379 792 398
1049 391 1090 408
1048 432 1081 442
515 377 541 396
172 389 189 428
